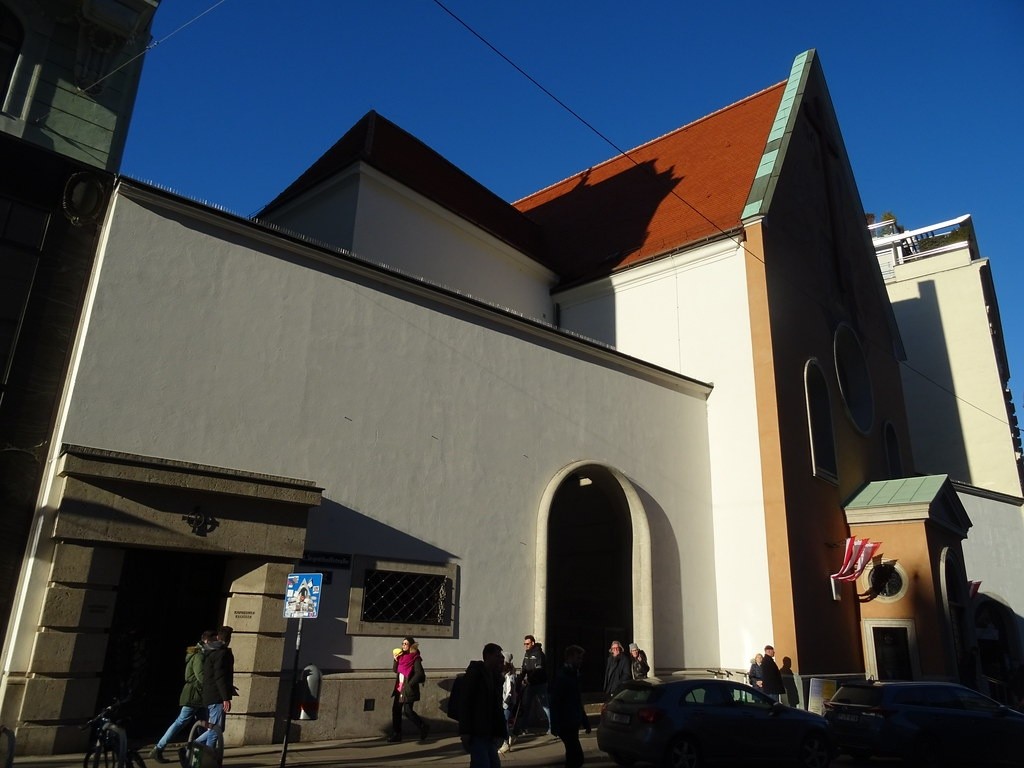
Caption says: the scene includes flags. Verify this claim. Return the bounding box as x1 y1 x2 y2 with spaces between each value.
831 536 882 601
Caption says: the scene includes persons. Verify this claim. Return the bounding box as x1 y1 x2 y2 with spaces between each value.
149 632 220 764
749 654 764 703
547 644 591 768
176 628 239 768
1010 663 1024 708
511 637 551 744
629 643 650 679
387 637 429 742
497 663 518 755
761 646 786 703
460 644 508 768
604 641 633 706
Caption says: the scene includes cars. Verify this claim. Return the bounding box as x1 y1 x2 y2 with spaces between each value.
597 675 839 768
821 680 1024 768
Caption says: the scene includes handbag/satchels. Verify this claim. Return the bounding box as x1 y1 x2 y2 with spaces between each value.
412 671 426 683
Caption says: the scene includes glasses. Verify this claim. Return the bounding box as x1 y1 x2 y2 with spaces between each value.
523 642 531 645
401 643 408 645
612 647 619 651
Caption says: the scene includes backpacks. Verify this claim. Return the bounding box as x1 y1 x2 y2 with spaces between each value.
447 674 470 724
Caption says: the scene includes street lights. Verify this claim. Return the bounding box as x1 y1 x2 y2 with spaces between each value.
0 171 106 412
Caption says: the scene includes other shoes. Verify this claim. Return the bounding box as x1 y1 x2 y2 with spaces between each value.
388 735 401 742
420 725 430 740
178 747 186 768
148 746 170 764
498 743 509 754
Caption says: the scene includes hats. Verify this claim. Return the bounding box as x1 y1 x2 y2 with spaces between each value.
609 641 624 653
501 652 513 663
629 644 640 653
765 645 774 650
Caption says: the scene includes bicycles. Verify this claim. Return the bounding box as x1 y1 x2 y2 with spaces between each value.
79 699 137 768
